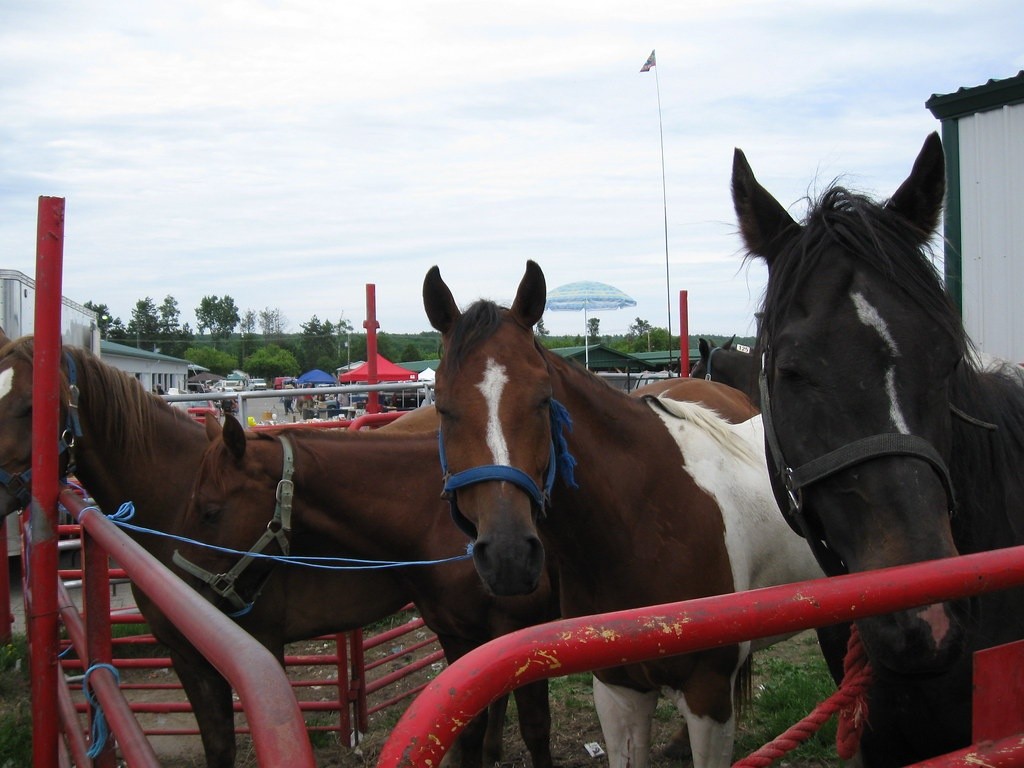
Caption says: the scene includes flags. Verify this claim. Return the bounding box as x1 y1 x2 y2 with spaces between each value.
638 48 655 72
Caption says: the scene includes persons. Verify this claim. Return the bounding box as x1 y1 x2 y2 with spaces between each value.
149 381 241 421
283 381 348 415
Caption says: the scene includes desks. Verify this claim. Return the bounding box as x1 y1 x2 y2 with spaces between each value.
319 408 349 419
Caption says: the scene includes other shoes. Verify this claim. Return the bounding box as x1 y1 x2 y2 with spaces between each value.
292 411 294 414
285 413 288 415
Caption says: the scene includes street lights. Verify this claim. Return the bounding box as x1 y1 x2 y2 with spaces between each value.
345 339 352 407
646 330 651 352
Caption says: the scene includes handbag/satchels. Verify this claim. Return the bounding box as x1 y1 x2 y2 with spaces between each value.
279 397 285 403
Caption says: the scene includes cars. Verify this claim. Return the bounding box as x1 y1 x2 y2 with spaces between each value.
167 400 220 426
273 377 290 390
322 394 368 410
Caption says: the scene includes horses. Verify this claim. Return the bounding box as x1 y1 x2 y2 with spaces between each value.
0 132 1024 768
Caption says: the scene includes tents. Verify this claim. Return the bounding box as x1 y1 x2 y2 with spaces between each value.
187 371 252 396
297 352 436 411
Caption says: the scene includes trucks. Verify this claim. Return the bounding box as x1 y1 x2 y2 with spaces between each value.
209 380 243 390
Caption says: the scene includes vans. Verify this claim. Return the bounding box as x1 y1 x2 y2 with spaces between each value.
248 379 267 391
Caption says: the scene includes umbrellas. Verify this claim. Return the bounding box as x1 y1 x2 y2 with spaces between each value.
188 364 210 373
544 280 638 371
